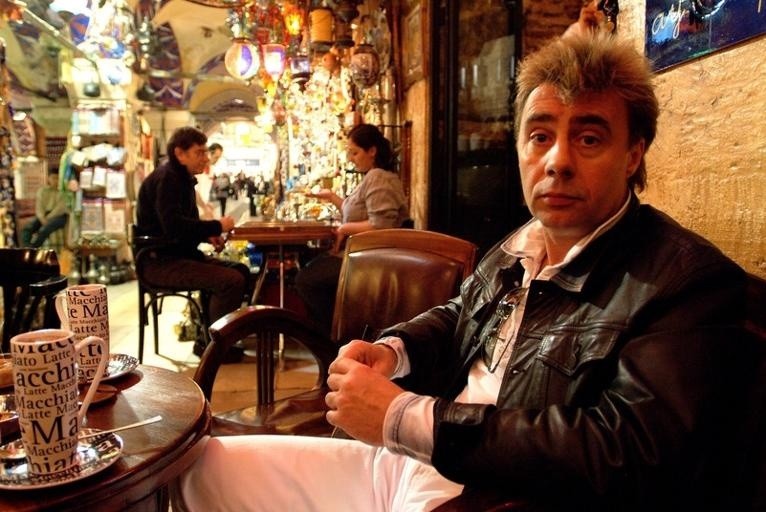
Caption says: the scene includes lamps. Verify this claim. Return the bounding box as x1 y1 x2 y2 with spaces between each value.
224 37 260 87
350 37 380 100
261 44 286 84
290 57 311 94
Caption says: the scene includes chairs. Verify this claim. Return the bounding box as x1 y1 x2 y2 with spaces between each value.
160 228 481 511
126 225 211 365
0 248 67 353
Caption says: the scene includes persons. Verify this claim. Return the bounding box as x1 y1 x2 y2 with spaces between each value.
210 166 273 217
193 142 223 220
293 123 410 348
135 126 248 364
18 167 68 250
165 24 766 511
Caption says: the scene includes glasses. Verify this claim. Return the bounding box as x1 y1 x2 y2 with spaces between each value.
481 285 532 374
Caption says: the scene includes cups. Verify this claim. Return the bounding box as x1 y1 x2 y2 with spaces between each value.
9 326 110 477
54 283 110 380
0 359 20 435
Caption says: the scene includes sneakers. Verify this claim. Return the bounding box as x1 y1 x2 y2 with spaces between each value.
192 334 245 366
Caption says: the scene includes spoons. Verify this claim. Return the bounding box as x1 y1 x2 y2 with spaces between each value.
1 412 164 462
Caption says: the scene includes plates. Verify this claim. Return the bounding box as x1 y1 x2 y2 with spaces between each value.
79 352 141 386
1 427 125 493
74 382 118 406
